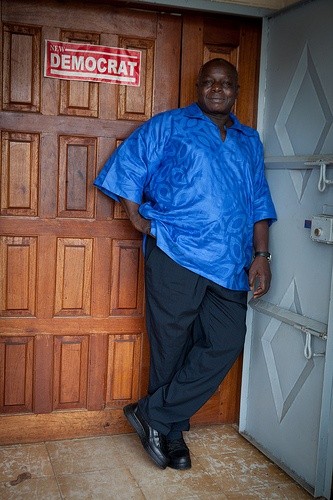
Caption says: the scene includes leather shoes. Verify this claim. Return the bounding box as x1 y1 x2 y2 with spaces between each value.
123 401 170 469
160 432 192 470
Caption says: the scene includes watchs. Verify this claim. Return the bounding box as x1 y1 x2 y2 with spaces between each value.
254 252 272 260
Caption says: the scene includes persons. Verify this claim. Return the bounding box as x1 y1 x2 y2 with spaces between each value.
93 53 278 470
